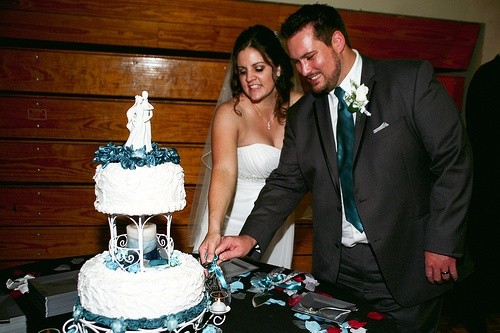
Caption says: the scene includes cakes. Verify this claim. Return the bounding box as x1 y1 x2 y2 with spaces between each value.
92 91 186 216
73 221 208 333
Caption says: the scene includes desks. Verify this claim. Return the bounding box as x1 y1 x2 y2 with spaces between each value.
0 253 399 333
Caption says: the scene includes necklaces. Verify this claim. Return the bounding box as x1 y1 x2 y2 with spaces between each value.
251 99 277 130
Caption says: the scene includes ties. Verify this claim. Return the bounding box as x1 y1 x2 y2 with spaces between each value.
333 86 365 234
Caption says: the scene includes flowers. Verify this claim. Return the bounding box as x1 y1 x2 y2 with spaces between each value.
343 79 372 116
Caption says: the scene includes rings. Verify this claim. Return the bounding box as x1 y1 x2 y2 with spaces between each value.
441 271 449 275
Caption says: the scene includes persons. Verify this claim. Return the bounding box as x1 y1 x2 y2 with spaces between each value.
124 91 154 154
213 3 480 333
464 51 499 303
191 24 295 268
